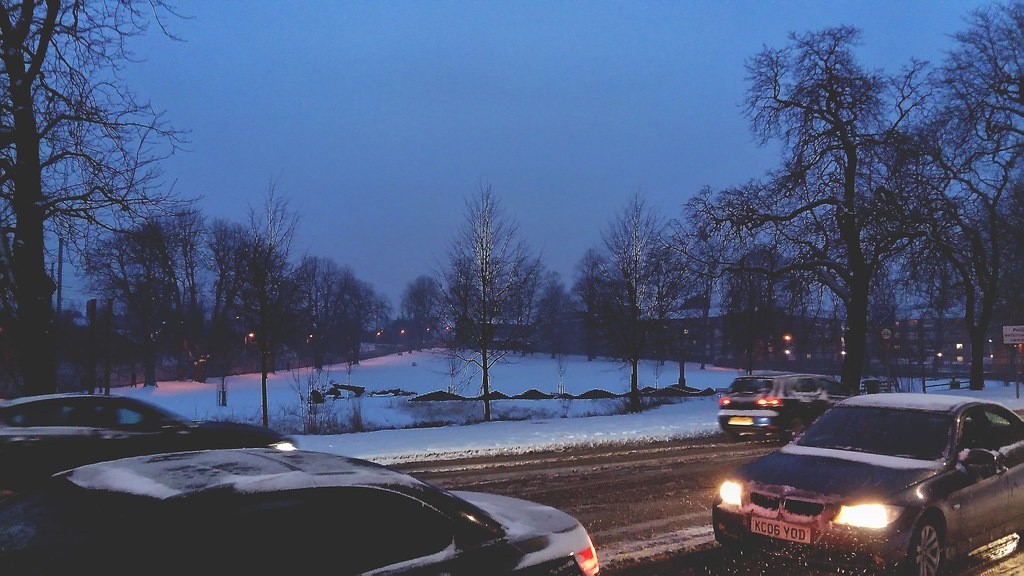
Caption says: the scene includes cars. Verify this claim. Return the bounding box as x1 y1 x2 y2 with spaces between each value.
0 447 600 576
718 374 855 444
711 392 1024 576
0 394 298 498
